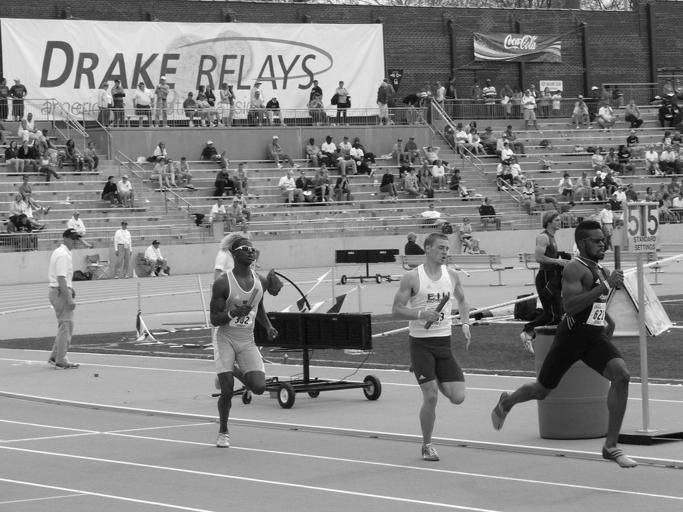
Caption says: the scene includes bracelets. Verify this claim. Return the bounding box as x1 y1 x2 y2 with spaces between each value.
603 280 610 290
228 310 233 320
462 322 471 326
418 310 421 318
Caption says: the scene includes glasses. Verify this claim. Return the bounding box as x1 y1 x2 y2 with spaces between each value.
235 245 256 252
592 238 605 243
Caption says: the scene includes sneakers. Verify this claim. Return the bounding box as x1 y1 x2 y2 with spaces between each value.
216 433 230 447
150 272 156 276
422 443 440 461
520 332 535 354
602 446 637 467
158 272 168 277
48 358 80 370
492 392 510 430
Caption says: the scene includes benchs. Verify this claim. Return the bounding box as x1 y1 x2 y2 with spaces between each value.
0 105 683 288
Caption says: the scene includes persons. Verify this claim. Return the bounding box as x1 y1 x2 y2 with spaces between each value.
392 233 472 462
518 78 683 250
490 219 638 468
210 239 278 448
130 76 376 234
0 78 134 253
48 228 80 370
112 221 169 279
376 79 528 255
520 210 571 354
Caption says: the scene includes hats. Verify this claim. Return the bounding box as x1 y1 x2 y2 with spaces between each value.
63 228 82 239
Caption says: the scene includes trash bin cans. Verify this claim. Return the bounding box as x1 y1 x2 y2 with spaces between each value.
534 325 612 440
